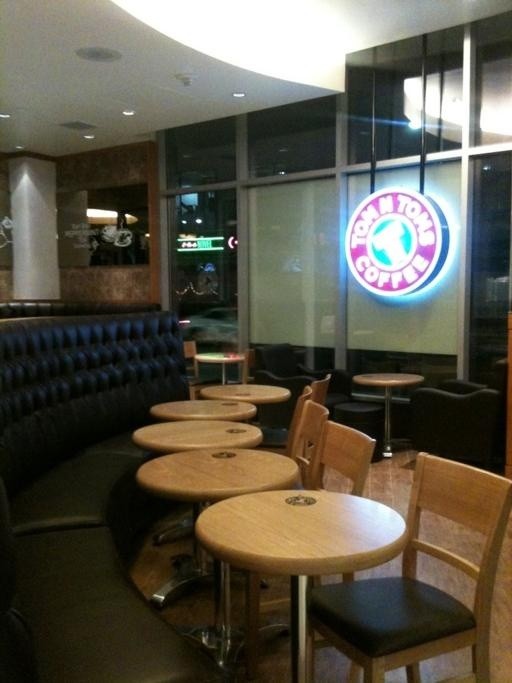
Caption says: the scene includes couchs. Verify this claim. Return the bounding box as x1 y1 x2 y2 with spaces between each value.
0 526 236 678
0 301 194 535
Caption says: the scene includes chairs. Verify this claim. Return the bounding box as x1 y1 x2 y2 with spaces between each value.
141 338 512 678
